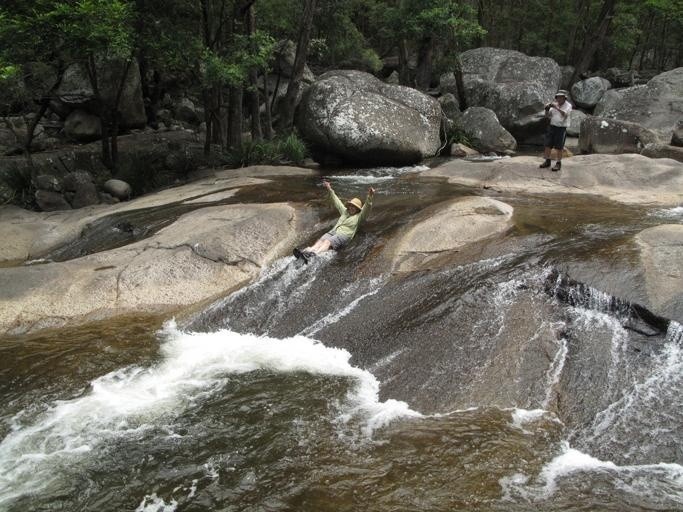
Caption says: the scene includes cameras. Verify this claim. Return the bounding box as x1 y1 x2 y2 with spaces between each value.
545 104 553 111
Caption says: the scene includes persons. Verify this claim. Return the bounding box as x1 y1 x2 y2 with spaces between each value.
538 89 573 172
292 181 376 264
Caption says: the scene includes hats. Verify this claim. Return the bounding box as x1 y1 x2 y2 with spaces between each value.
344 198 362 210
555 89 569 100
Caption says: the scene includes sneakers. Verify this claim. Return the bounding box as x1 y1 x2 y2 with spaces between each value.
540 162 551 167
293 248 316 264
553 163 561 171
